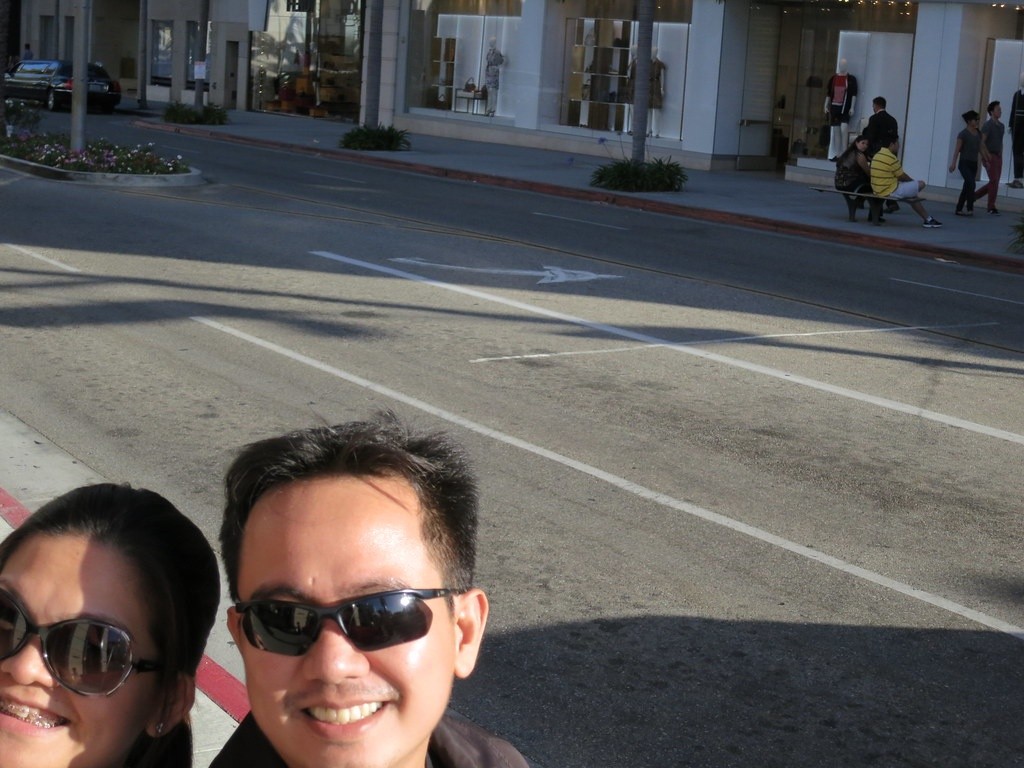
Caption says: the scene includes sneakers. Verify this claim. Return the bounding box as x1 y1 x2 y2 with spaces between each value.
923 216 943 227
987 209 1001 216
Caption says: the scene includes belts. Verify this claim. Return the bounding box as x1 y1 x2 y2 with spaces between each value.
649 76 659 81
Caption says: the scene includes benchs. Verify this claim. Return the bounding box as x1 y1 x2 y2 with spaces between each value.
809 186 926 225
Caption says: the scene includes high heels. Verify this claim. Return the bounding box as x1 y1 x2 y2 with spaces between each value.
655 135 659 138
646 132 652 137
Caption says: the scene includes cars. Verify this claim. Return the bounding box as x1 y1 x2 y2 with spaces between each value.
1 58 122 112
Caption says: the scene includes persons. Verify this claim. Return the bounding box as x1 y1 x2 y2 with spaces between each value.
484 37 504 117
625 45 664 137
823 59 857 161
1006 72 1024 188
870 131 943 227
206 418 531 768
863 97 900 213
0 484 221 768
834 136 885 222
22 43 34 60
949 100 1005 216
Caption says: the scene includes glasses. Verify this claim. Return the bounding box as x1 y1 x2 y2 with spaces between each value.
235 589 465 657
973 118 980 122
0 588 182 698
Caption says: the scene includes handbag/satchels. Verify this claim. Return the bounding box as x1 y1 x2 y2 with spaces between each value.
791 138 808 155
585 27 596 46
806 75 823 88
465 77 476 92
613 38 631 49
582 79 591 101
819 112 830 147
481 85 487 98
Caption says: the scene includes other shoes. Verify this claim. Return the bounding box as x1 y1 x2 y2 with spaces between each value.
955 210 973 216
884 205 899 213
829 156 839 162
628 130 633 136
484 111 494 117
857 204 864 209
1006 180 1024 188
